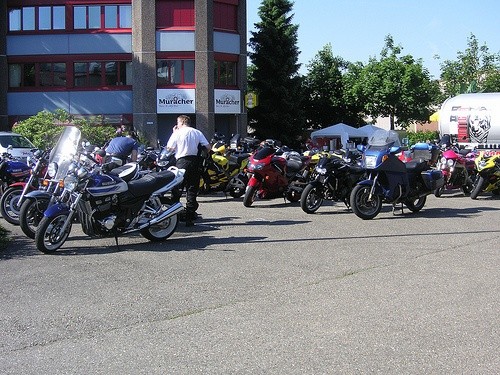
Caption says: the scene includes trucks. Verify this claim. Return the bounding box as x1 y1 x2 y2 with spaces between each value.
438 92 499 151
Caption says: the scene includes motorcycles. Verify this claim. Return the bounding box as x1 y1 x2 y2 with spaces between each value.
33 153 186 254
0 125 176 239
197 133 367 214
396 134 500 200
349 131 445 220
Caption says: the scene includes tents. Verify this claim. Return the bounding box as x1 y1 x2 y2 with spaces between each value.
309 123 393 149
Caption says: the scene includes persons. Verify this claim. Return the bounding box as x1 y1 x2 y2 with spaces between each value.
104 130 140 165
116 126 127 137
164 115 211 222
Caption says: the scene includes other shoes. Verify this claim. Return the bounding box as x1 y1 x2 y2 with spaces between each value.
185 221 194 227
